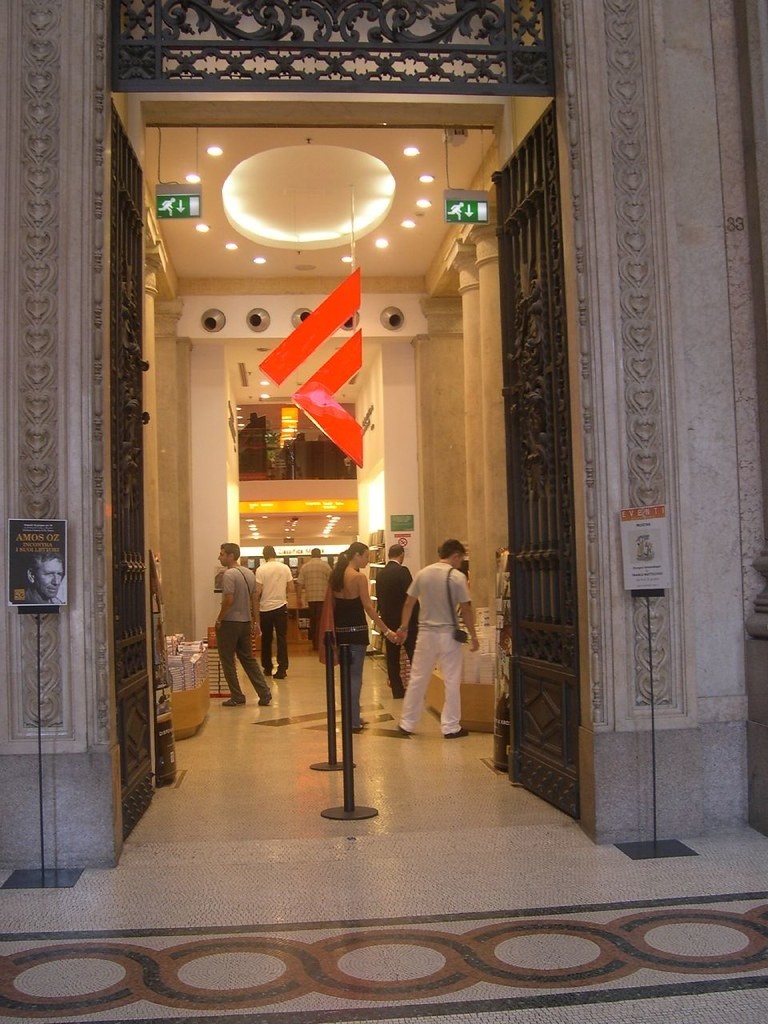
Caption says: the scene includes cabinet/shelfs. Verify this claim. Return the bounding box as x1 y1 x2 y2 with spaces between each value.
367 546 386 636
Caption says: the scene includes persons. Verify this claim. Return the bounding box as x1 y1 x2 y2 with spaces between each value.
12 552 66 604
296 549 332 640
331 542 396 731
256 546 294 678
377 543 420 699
396 539 480 738
215 543 272 706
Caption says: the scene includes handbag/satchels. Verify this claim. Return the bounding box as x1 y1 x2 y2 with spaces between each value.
317 570 339 666
453 629 468 644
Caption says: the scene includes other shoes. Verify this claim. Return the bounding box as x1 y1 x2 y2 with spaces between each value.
262 669 272 676
222 695 246 706
258 693 272 705
272 670 288 679
394 688 406 699
352 722 364 733
444 728 469 738
397 725 413 735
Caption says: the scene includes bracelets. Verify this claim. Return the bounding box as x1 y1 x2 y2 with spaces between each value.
384 629 392 637
398 625 408 633
216 621 221 624
